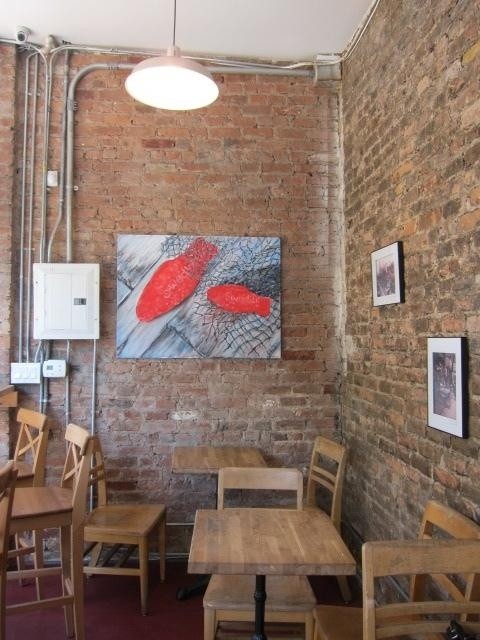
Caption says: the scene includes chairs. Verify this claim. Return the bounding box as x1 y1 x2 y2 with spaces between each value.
65 437 168 618
5 422 96 640
311 499 480 640
1 458 19 639
360 538 480 640
7 408 53 600
200 466 321 639
259 435 353 603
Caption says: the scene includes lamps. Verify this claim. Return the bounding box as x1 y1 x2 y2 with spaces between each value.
124 1 220 111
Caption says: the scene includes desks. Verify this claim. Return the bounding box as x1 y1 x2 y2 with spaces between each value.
171 445 271 601
184 506 359 640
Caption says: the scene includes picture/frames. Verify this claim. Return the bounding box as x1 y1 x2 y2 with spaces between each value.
424 335 470 440
369 240 406 308
